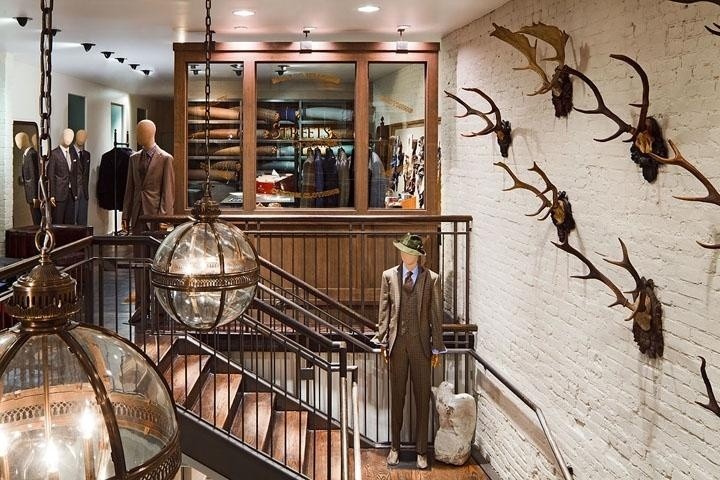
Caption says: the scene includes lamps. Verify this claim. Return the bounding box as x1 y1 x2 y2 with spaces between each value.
150 1 263 337
0 0 183 480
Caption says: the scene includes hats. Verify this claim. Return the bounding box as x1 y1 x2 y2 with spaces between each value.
392 233 427 256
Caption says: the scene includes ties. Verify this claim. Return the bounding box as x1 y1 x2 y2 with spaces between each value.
79 151 83 163
65 150 71 171
405 270 414 291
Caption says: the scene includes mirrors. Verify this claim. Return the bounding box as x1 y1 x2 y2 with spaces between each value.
12 120 42 229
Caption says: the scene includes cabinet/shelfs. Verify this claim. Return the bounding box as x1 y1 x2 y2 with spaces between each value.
188 96 362 185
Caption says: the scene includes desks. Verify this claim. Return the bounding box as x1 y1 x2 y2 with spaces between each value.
220 193 294 206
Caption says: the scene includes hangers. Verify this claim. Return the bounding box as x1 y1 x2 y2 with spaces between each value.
301 138 377 159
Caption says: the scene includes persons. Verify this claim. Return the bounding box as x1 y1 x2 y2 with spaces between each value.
379 236 446 469
15 128 89 227
122 120 175 327
